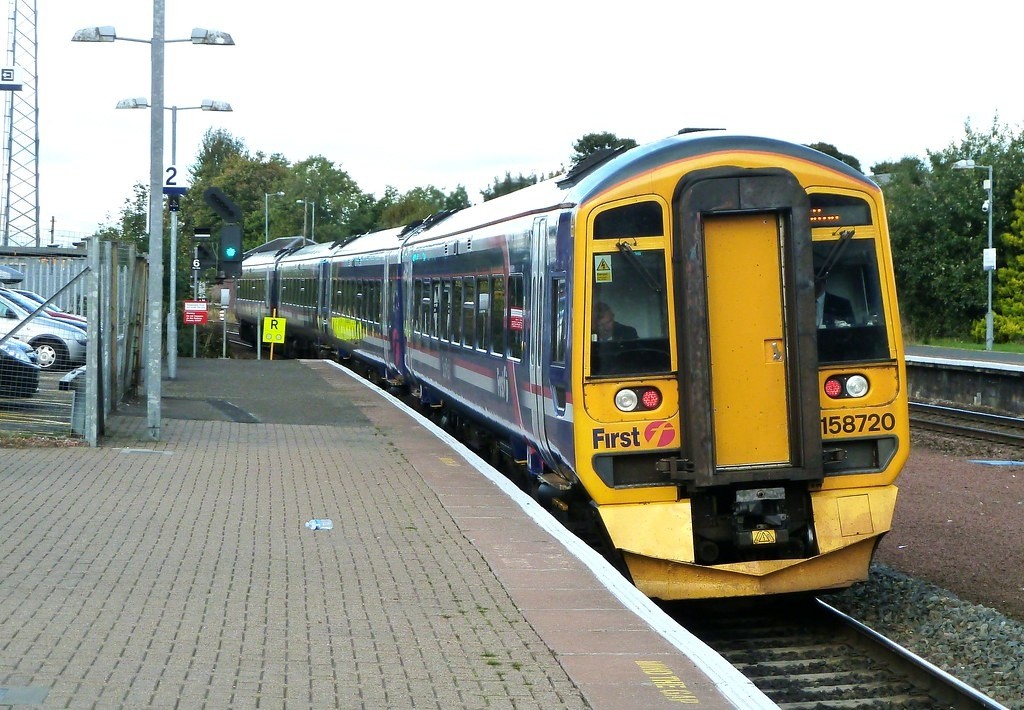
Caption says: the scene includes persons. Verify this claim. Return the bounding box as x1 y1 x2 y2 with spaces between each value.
590 303 640 343
812 269 859 329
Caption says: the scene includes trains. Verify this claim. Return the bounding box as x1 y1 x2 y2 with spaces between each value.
229 124 910 609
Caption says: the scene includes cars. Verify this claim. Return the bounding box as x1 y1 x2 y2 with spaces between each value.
6 292 88 332
5 287 87 323
0 337 42 398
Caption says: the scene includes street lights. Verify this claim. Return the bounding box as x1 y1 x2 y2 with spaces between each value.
296 198 318 242
952 159 996 349
265 191 286 244
73 0 238 442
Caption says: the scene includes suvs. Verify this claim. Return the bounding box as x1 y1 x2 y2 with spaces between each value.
0 265 90 369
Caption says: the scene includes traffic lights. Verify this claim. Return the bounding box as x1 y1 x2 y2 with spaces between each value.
218 223 244 278
